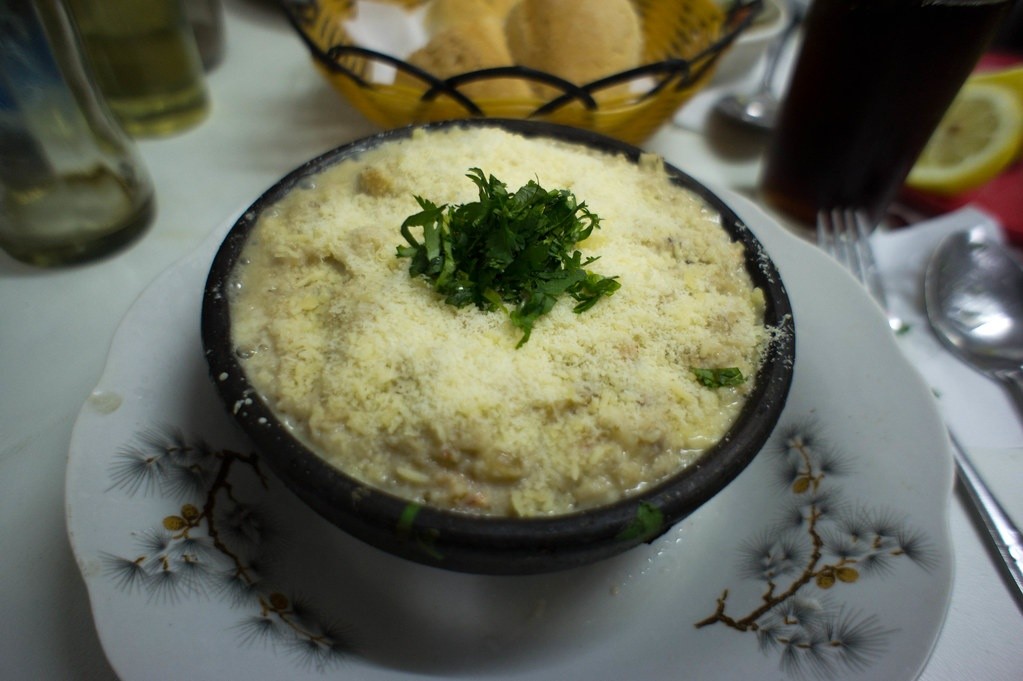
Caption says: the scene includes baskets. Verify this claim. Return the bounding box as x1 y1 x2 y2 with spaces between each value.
283 0 765 149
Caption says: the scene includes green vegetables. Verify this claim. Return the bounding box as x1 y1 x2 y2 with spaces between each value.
393 160 746 542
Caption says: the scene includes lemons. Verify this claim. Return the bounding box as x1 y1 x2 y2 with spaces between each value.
902 80 1022 200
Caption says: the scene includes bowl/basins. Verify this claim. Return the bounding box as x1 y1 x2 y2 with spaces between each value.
200 117 797 576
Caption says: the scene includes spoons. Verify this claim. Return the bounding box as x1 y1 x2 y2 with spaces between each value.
716 0 803 131
924 223 1023 413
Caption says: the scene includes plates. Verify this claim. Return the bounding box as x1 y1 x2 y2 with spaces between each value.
64 158 957 681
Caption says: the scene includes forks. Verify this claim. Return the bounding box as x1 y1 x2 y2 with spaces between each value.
817 207 1023 612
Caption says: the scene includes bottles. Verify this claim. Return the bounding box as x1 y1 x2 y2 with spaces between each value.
0 0 224 269
757 0 1023 236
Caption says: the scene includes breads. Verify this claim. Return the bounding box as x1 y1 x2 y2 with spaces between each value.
390 0 645 113
